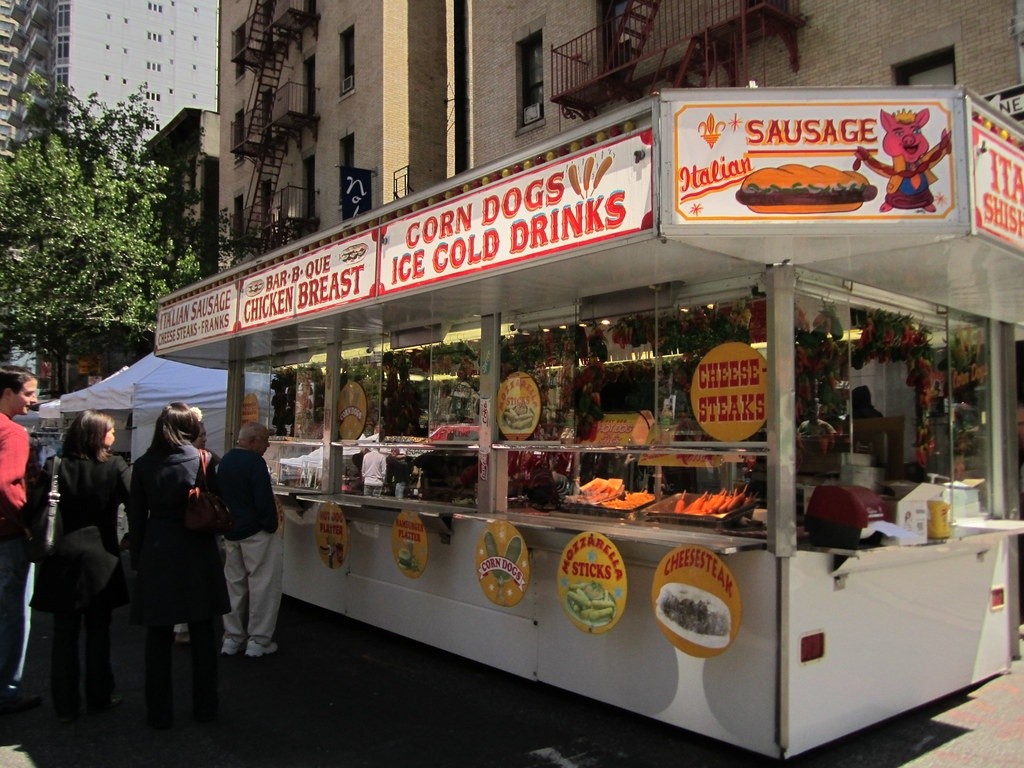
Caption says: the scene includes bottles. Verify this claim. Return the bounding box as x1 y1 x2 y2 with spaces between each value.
566 409 575 429
408 465 423 500
660 404 672 445
299 459 323 489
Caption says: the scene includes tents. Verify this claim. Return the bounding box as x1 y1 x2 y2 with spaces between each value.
12 352 292 463
280 433 366 490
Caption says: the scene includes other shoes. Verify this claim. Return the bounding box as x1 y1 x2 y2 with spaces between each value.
0 693 42 713
193 711 216 721
83 695 122 714
145 714 175 729
175 632 190 643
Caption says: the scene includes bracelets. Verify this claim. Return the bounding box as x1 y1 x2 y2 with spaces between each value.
24 534 33 541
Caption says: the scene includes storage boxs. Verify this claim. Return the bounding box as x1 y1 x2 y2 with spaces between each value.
804 477 987 545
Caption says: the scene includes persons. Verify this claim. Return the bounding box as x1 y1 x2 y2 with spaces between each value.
25 409 131 722
0 365 45 716
797 405 835 478
843 385 886 463
353 447 410 499
214 422 284 658
129 403 221 729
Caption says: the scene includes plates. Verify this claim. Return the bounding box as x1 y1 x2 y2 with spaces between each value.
564 600 616 627
655 583 731 648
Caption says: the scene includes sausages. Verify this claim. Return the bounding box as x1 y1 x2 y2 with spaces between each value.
504 409 533 429
713 493 750 513
567 583 614 620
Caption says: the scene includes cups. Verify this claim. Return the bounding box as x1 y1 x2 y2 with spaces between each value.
336 543 343 564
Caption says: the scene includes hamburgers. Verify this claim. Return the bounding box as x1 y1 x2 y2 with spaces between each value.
655 582 731 649
397 549 410 568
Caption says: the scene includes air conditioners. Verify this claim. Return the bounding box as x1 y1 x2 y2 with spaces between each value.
343 76 354 94
524 102 544 125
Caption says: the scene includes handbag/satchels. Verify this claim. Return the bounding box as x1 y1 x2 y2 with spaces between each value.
184 448 234 533
26 455 61 561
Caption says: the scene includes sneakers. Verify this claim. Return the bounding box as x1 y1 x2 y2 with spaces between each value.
245 638 280 657
220 636 246 656
